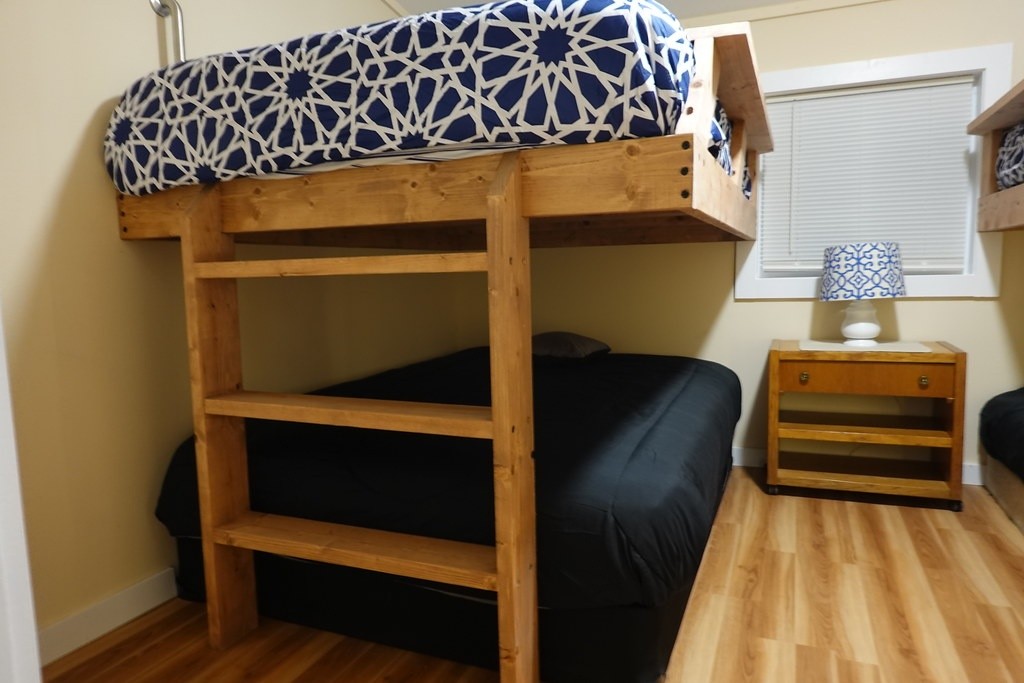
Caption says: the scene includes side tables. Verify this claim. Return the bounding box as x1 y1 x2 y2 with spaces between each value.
765 336 970 512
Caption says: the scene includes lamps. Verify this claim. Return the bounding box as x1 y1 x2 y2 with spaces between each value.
814 236 917 344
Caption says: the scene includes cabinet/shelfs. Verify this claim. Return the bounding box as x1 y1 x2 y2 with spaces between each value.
963 77 1024 232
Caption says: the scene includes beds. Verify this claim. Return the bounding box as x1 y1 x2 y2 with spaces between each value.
95 0 777 252
155 335 746 683
980 380 1024 535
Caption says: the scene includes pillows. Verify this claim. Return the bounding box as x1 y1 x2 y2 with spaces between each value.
533 328 610 359
